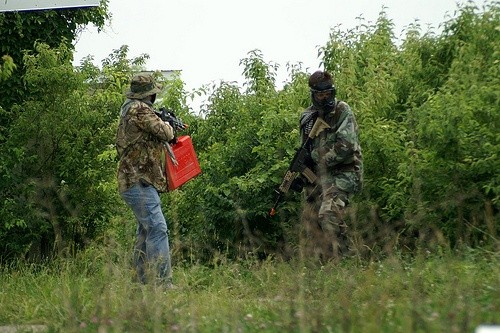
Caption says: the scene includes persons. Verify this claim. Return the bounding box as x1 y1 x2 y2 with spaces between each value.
114 72 189 297
289 71 364 272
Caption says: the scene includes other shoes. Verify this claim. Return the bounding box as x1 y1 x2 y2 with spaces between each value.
154 279 180 292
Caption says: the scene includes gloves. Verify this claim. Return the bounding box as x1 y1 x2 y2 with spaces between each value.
290 177 304 193
169 137 177 144
302 152 314 168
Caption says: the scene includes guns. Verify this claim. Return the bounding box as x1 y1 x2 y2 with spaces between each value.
270 117 331 217
152 105 189 145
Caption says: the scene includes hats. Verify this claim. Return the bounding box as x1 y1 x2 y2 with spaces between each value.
128 74 158 99
